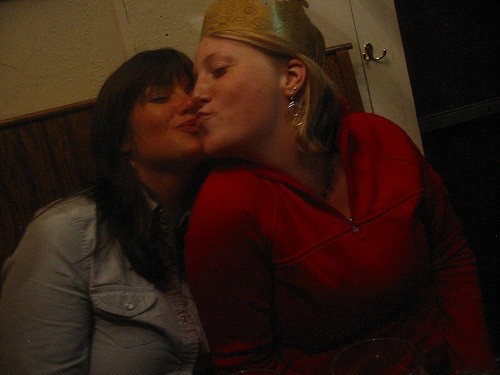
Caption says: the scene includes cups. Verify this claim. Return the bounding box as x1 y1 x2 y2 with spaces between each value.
330 338 429 375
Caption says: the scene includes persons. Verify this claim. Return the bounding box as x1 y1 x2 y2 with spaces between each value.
182 0 496 375
0 47 212 375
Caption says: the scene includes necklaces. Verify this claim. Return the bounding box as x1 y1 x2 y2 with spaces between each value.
313 162 336 200
